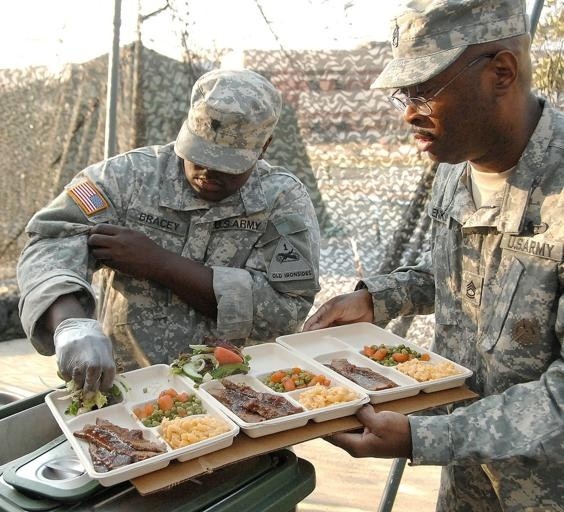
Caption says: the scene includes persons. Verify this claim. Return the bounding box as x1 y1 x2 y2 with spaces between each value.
304 1 564 512
17 69 321 395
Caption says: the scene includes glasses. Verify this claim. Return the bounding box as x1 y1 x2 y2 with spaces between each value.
388 54 494 116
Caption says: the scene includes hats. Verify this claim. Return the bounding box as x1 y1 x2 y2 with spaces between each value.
174 67 282 175
370 0 529 89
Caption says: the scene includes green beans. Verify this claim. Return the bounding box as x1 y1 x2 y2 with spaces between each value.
369 344 417 366
141 393 207 428
265 371 310 393
